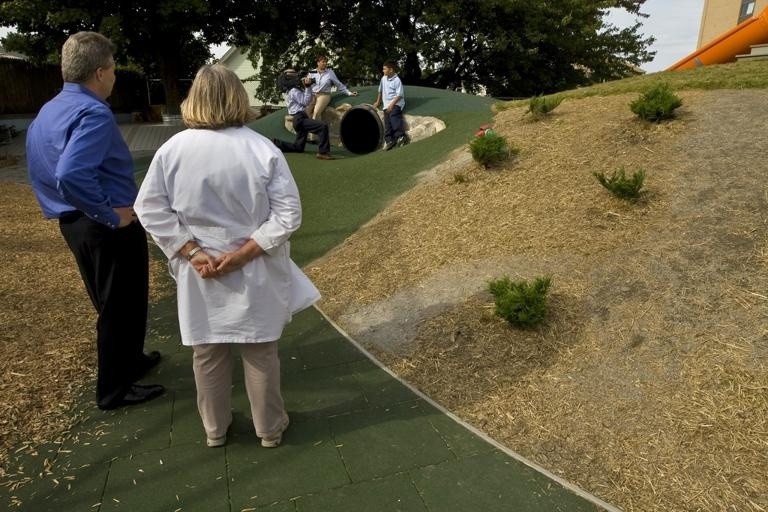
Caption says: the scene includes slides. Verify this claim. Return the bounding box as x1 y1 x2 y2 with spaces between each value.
338 5 768 155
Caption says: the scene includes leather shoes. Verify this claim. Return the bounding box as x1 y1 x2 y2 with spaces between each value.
143 351 161 371
316 153 335 160
115 384 165 409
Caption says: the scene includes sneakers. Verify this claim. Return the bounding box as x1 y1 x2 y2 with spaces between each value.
206 413 233 448
261 411 290 448
383 140 396 151
397 136 407 147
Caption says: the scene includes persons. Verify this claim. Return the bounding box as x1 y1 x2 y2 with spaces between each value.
23 31 165 409
133 64 322 448
306 54 357 141
372 59 408 151
272 69 335 160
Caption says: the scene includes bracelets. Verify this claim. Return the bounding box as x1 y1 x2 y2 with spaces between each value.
187 247 202 260
